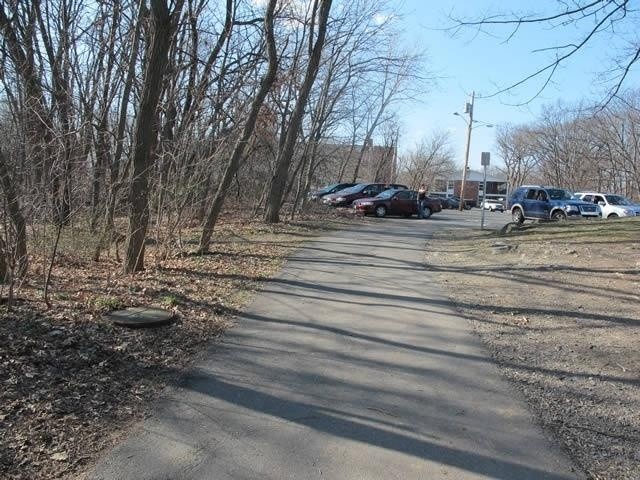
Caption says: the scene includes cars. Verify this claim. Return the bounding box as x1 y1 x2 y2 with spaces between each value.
312 183 472 220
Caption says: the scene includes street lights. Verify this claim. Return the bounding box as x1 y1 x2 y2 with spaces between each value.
453 110 493 211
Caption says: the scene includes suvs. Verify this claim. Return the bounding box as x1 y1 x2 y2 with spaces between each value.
481 199 505 213
508 184 640 225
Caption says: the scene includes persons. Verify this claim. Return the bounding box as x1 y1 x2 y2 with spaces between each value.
537 192 545 201
416 183 427 220
